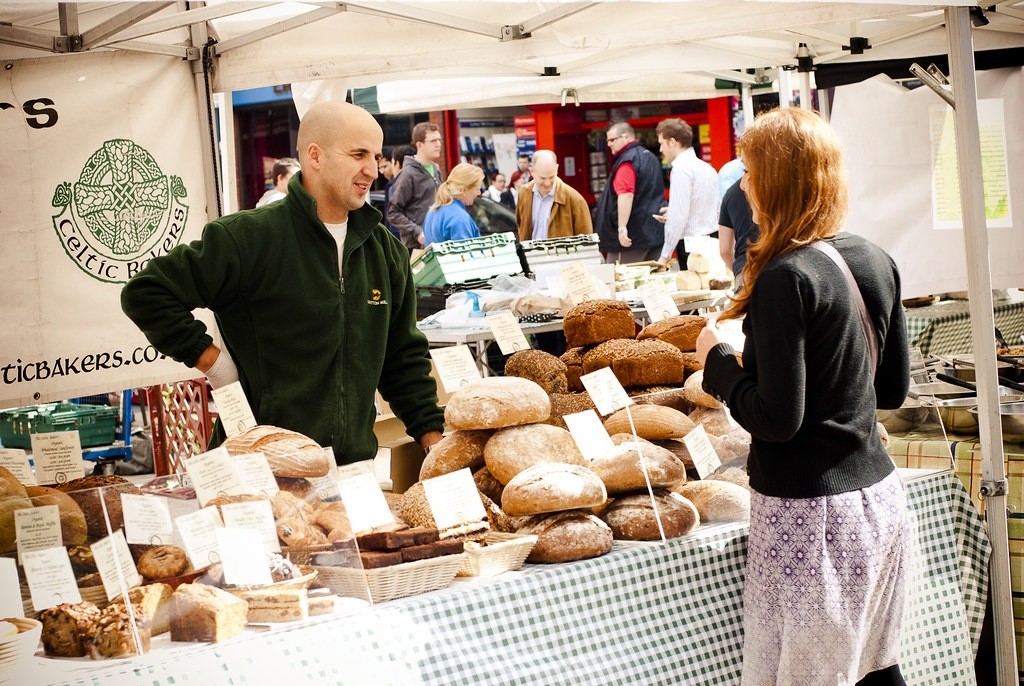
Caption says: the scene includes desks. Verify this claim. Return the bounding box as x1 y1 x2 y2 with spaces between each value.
903 293 1023 357
418 294 733 378
14 468 991 686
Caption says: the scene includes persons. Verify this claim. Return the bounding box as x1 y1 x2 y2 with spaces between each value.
696 105 920 686
718 158 764 294
378 121 534 256
120 100 446 468
595 121 668 263
652 118 721 270
256 157 302 209
514 150 594 242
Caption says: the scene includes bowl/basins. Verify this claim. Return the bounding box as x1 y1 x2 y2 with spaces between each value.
874 354 1024 444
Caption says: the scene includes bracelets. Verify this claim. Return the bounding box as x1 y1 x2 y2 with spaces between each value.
424 444 436 455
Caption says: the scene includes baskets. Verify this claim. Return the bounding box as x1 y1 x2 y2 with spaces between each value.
20 566 110 612
296 552 467 604
0 616 43 683
282 543 336 590
456 531 540 579
19 592 44 618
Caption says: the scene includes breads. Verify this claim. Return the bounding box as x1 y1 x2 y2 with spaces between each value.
401 299 751 564
677 252 710 290
0 424 465 661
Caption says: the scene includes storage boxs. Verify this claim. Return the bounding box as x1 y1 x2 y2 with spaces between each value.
411 231 601 317
0 403 120 449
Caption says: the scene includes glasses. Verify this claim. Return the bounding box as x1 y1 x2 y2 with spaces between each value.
605 133 625 142
424 138 443 143
378 165 387 174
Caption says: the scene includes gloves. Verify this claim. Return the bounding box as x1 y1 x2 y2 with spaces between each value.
205 350 240 390
